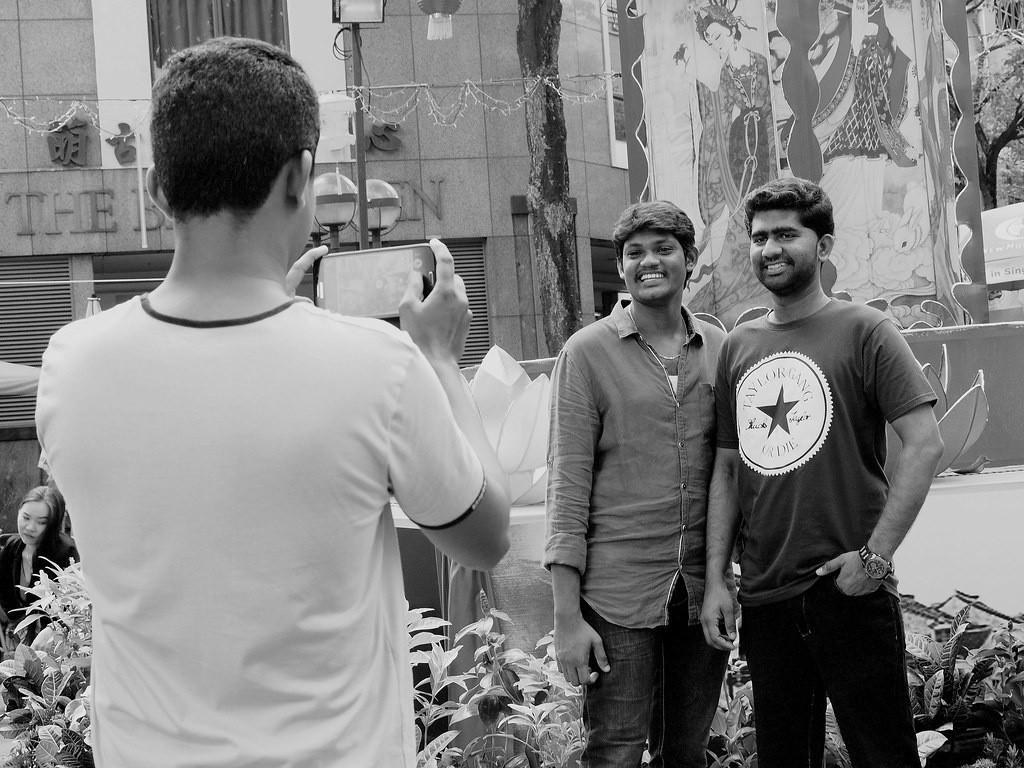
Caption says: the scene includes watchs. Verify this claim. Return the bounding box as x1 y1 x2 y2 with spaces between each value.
860 545 893 581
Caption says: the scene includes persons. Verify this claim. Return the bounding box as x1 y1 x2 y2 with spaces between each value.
544 199 739 768
699 178 945 768
0 486 79 662
35 37 510 768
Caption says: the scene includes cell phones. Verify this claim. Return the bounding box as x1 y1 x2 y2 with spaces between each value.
312 242 436 322
